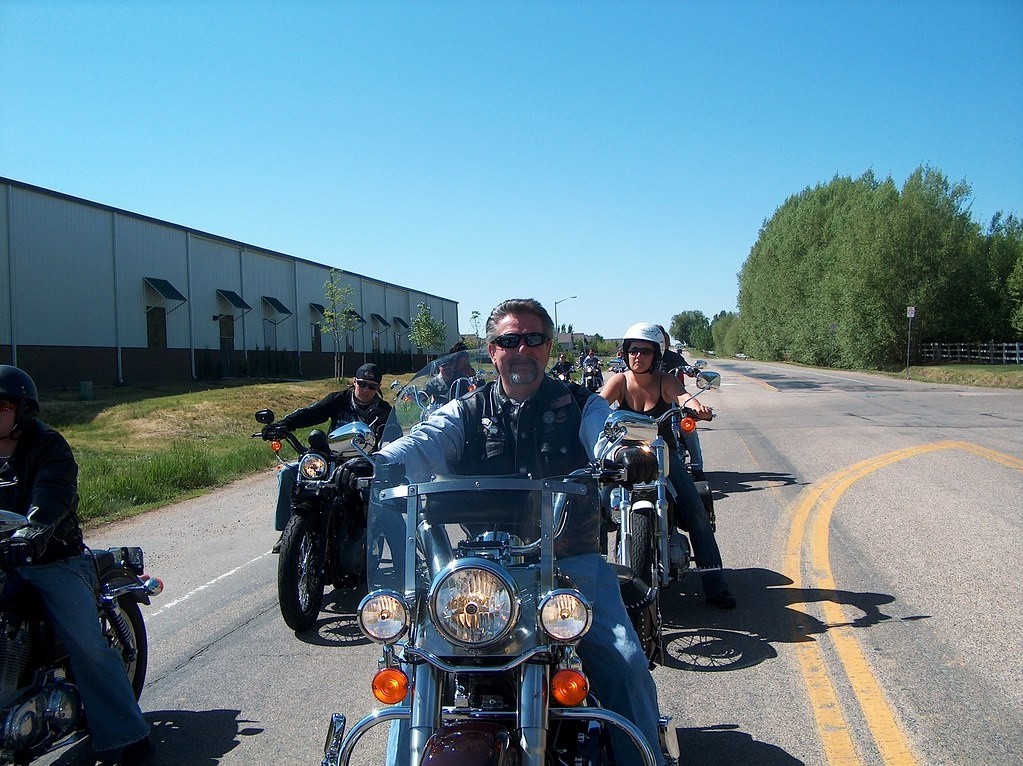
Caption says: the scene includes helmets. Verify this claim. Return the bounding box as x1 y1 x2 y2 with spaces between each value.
621 323 666 360
0 365 40 441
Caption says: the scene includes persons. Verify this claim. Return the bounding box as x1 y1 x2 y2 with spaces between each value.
548 347 623 387
261 364 425 627
405 341 485 422
329 298 666 766
595 323 736 611
0 365 150 766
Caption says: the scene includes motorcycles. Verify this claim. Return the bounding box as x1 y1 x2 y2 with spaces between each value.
576 360 605 392
321 349 680 766
600 371 721 591
607 358 628 373
251 408 385 631
547 366 577 382
390 369 486 422
0 476 164 766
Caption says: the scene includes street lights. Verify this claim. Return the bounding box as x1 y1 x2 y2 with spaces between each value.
555 296 577 361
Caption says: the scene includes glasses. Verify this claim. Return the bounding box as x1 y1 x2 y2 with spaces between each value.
629 347 655 356
441 364 454 368
490 332 550 348
356 380 379 390
0 400 17 412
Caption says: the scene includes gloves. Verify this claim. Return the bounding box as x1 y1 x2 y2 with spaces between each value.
327 457 384 495
0 537 35 574
260 422 282 439
615 447 660 492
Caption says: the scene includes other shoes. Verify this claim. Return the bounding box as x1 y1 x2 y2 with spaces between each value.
704 576 738 609
112 741 156 766
693 469 705 482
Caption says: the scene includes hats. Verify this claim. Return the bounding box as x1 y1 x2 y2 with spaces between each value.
356 363 382 384
437 353 454 364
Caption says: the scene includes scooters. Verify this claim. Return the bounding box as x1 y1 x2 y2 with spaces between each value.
490 369 499 380
